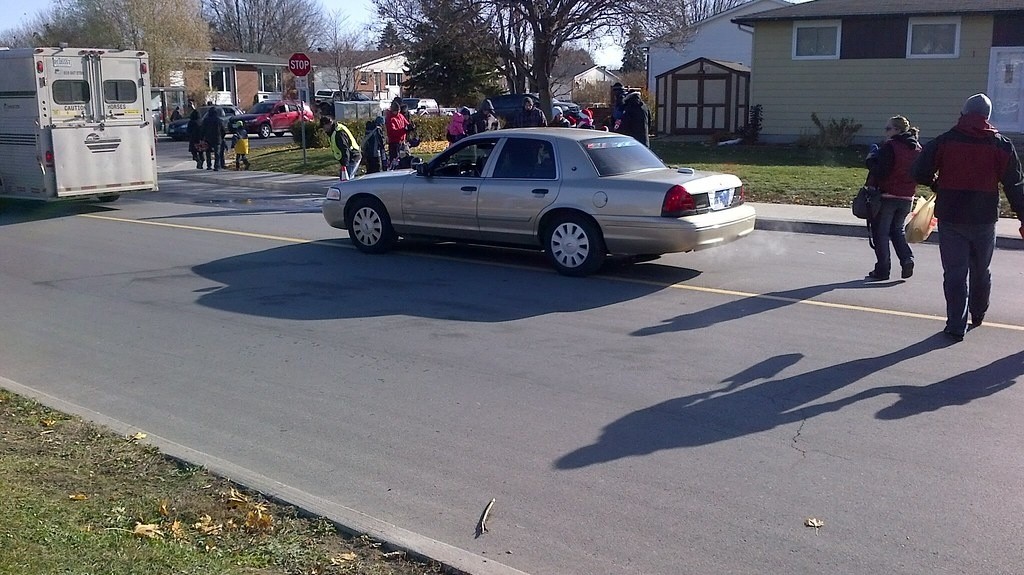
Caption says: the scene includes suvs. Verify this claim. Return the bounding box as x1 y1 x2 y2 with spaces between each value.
228 99 314 139
315 89 372 116
479 94 580 124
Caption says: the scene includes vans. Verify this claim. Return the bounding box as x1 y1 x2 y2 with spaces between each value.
402 98 439 115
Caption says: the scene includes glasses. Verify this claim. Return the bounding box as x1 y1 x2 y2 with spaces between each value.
886 126 898 131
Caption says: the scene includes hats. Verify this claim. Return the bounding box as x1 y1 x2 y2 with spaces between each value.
391 101 400 112
462 109 470 116
479 99 495 111
961 93 993 120
552 105 562 120
235 120 244 129
315 116 331 128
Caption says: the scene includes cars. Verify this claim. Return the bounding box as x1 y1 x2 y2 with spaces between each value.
323 127 756 277
167 104 244 139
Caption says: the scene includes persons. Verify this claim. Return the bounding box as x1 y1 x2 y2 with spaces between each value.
231 121 251 171
170 106 182 122
185 100 228 171
865 115 922 280
910 94 1024 339
317 82 652 181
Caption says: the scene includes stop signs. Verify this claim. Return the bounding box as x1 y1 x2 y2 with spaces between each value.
288 53 312 76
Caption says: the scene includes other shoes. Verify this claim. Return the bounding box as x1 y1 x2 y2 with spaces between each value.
244 162 250 170
206 168 211 170
233 168 239 171
213 167 218 170
943 324 963 342
221 165 225 169
972 313 984 325
196 158 205 169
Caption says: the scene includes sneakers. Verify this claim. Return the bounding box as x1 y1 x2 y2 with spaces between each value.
868 269 889 280
902 258 914 278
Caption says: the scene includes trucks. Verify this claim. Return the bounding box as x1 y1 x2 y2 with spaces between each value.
0 42 159 205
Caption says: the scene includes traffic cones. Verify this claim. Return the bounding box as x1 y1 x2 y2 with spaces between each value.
340 166 349 180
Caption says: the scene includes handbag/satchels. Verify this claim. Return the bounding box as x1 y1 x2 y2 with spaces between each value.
904 193 938 244
852 158 881 219
